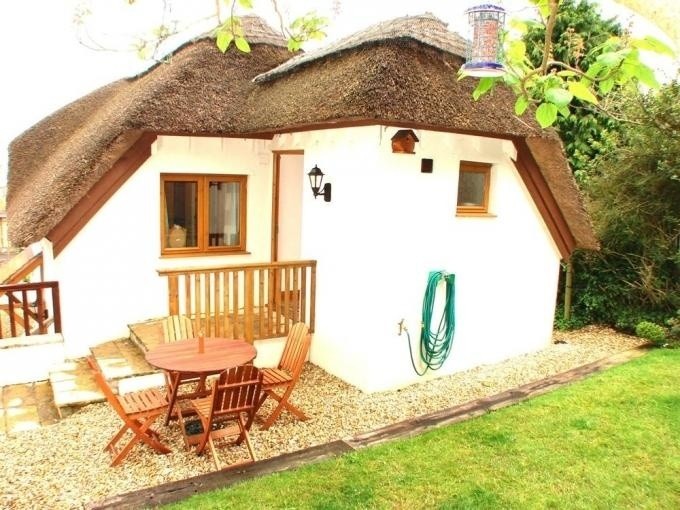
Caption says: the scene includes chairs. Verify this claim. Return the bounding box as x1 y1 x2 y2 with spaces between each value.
86 312 312 472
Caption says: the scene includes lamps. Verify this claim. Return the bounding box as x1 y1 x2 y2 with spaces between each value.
308 163 331 202
